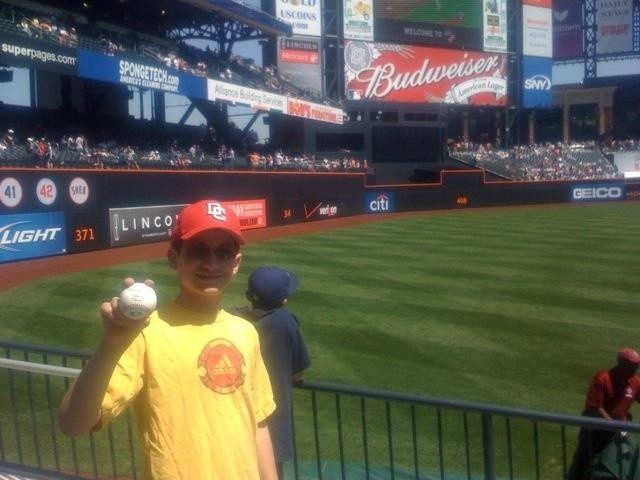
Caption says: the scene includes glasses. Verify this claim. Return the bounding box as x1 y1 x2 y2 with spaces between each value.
183 239 237 263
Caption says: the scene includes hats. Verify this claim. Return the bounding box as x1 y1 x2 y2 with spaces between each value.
248 266 298 301
171 199 245 247
617 348 640 366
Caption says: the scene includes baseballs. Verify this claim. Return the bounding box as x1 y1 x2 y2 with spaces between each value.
120 283 157 319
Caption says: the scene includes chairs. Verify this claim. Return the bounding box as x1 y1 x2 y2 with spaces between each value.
0 13 640 182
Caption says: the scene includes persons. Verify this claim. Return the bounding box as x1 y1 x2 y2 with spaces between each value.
56 198 281 479
565 347 640 480
232 264 309 479
446 136 640 183
1 125 370 172
14 14 332 109
